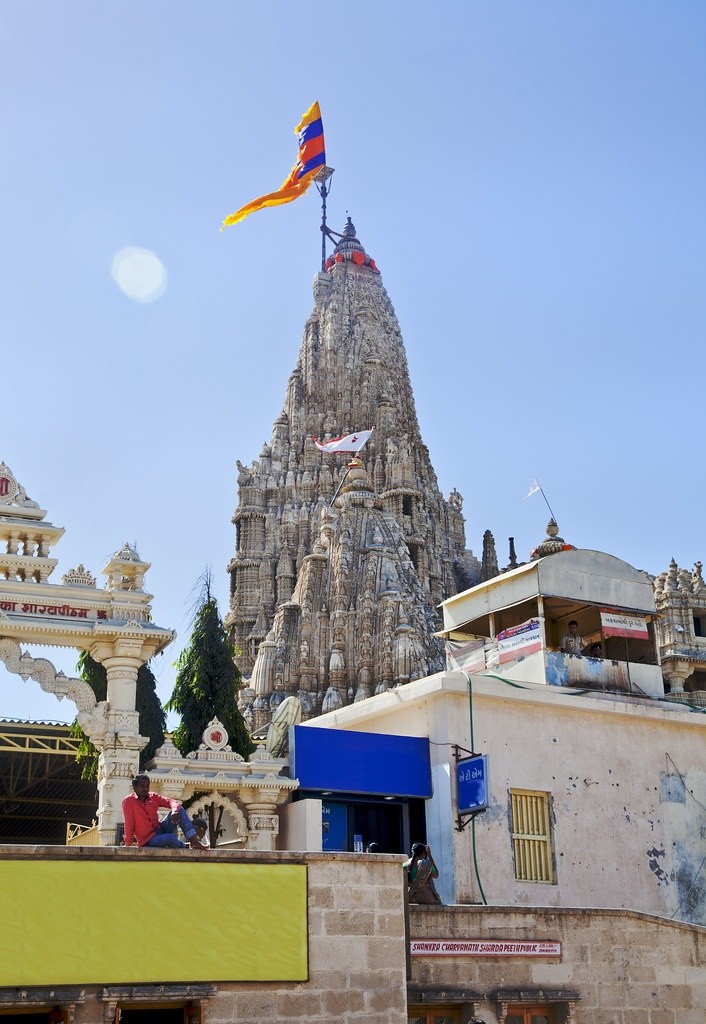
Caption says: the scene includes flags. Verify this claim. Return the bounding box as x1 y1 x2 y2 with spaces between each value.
527 479 540 496
312 430 371 454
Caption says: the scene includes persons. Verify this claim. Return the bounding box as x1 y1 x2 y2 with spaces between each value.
592 643 601 656
122 774 209 849
560 620 589 655
403 843 442 905
